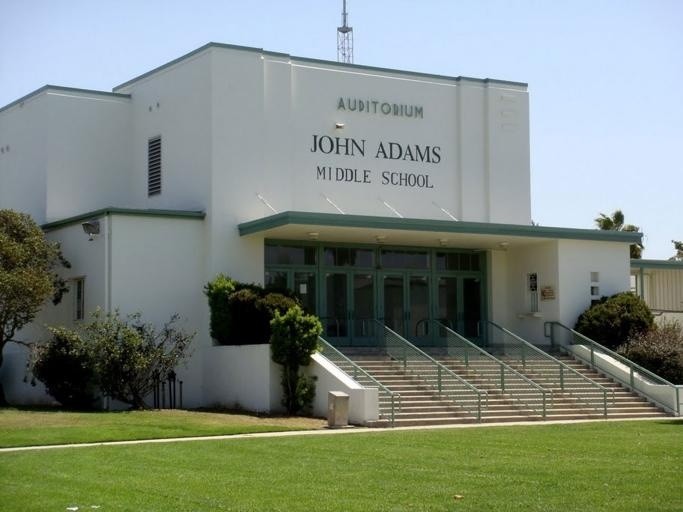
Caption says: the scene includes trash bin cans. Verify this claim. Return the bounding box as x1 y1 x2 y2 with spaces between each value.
327 390 349 428
